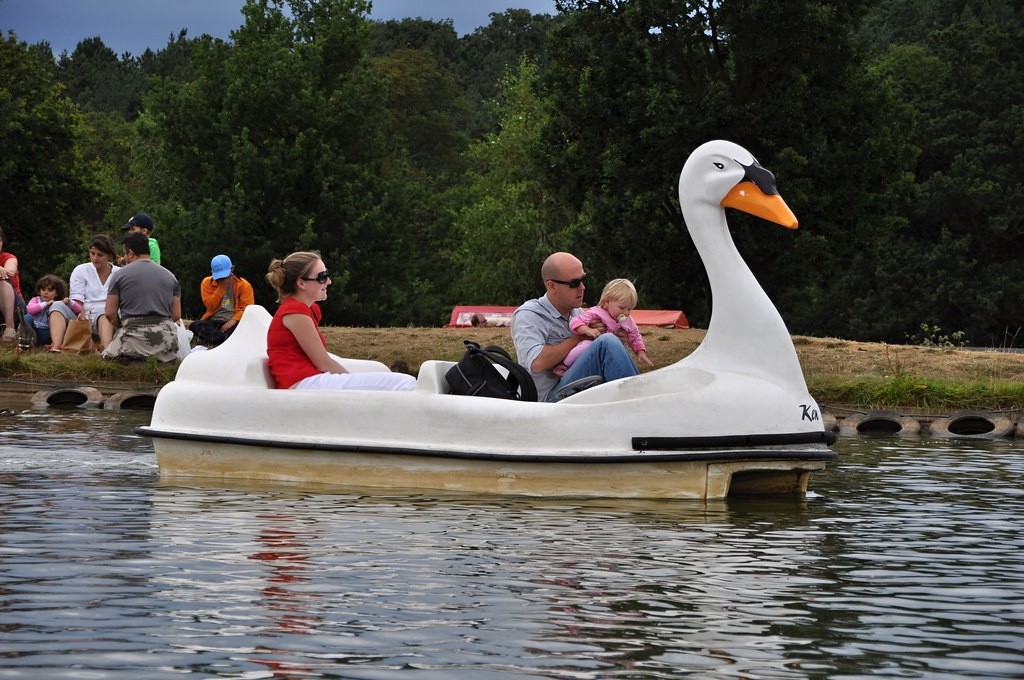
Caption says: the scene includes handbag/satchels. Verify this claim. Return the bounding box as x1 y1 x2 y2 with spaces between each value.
60 309 94 356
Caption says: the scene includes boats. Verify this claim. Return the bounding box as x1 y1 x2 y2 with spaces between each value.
132 138 841 504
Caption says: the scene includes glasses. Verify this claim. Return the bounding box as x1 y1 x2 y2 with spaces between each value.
546 273 588 288
302 270 331 284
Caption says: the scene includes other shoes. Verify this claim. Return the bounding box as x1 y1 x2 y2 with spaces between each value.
2 328 16 342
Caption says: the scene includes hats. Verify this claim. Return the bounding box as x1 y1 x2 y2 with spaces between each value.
120 214 154 229
211 254 233 280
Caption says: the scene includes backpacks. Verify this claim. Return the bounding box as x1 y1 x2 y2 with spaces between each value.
444 339 538 402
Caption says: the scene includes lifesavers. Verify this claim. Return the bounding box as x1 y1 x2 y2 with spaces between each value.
817 409 1023 447
28 381 159 411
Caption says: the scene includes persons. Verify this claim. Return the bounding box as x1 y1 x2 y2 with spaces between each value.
0 215 181 362
472 314 505 328
189 255 254 345
511 252 654 403
266 250 417 392
